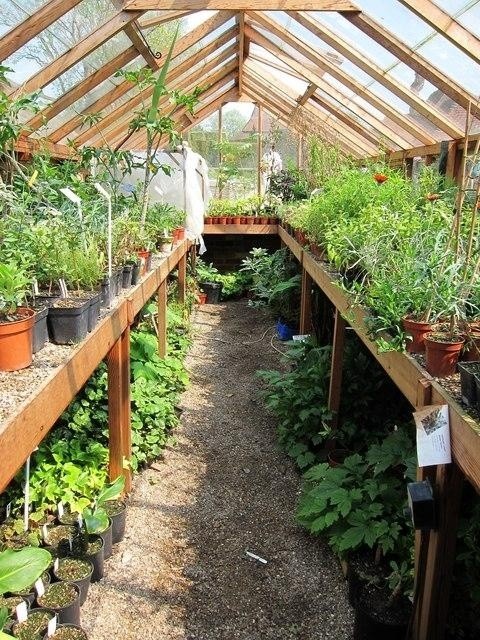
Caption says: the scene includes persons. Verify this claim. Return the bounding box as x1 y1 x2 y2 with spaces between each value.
261 145 282 195
